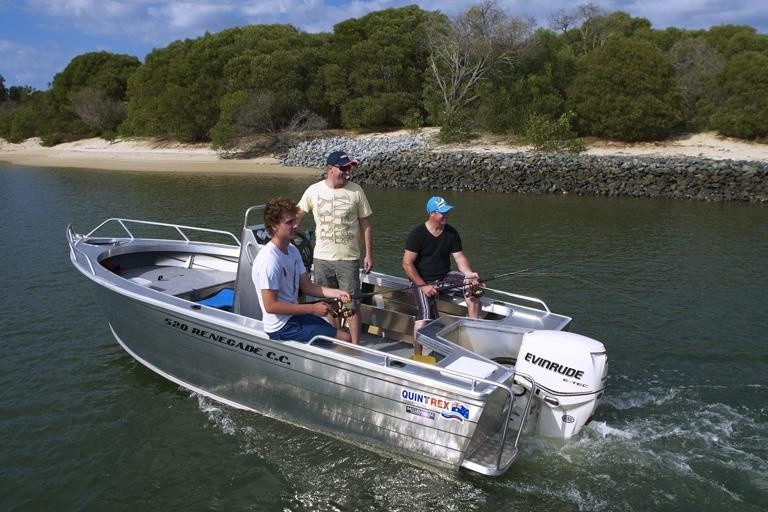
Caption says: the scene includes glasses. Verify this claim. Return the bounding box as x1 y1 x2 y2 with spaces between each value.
333 165 348 171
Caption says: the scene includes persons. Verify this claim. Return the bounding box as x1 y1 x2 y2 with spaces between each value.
293 150 374 347
251 196 353 356
402 195 487 356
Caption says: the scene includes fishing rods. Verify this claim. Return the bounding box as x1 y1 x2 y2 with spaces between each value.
301 262 650 320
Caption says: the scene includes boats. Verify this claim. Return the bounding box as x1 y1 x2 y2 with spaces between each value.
63 203 612 485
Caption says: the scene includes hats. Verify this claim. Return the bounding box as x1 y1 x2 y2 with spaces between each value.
327 151 357 167
426 196 454 214
